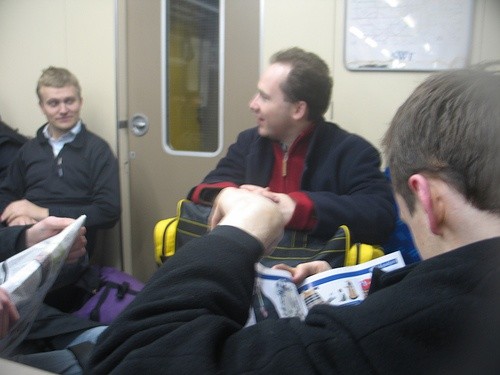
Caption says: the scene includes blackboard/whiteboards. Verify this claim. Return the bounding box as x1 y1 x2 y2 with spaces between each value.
343 0 475 72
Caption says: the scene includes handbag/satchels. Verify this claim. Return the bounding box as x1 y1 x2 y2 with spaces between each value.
154 200 384 269
76 267 145 323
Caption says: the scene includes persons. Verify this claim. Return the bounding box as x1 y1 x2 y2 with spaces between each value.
187 47 397 246
1 216 88 374
0 66 120 295
85 69 500 375
